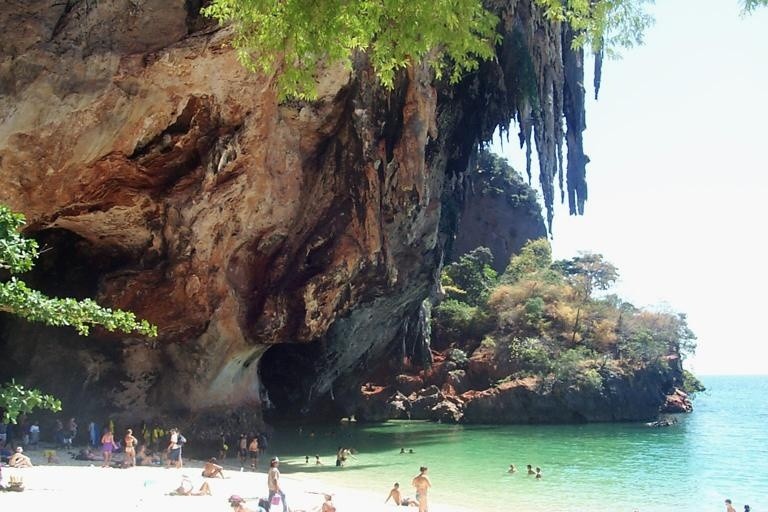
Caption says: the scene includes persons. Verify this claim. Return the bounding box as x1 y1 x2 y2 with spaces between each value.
321 493 336 512
412 466 432 512
527 465 535 474
536 468 541 478
79 449 97 461
744 505 750 512
136 425 187 469
228 495 257 512
97 426 120 468
385 483 419 507
267 456 290 512
507 465 517 472
124 429 137 467
1 408 40 467
409 449 413 453
400 448 405 453
306 454 323 464
53 417 78 450
173 476 213 496
202 457 226 479
336 447 357 467
216 432 259 473
85 417 97 451
726 499 736 512
